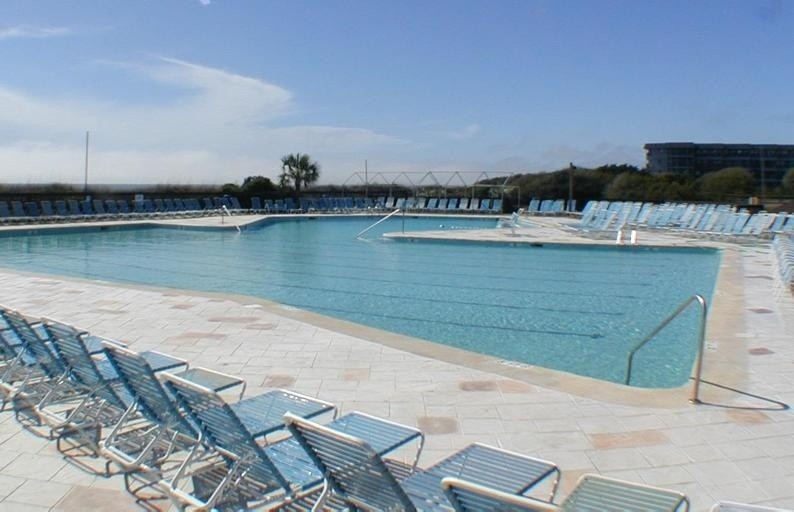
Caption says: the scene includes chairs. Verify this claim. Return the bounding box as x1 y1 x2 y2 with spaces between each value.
283 412 561 511
100 339 338 511
528 198 794 244
498 207 526 237
710 500 790 512
159 371 425 511
0 197 503 226
769 228 794 305
440 473 690 512
1 306 247 478
